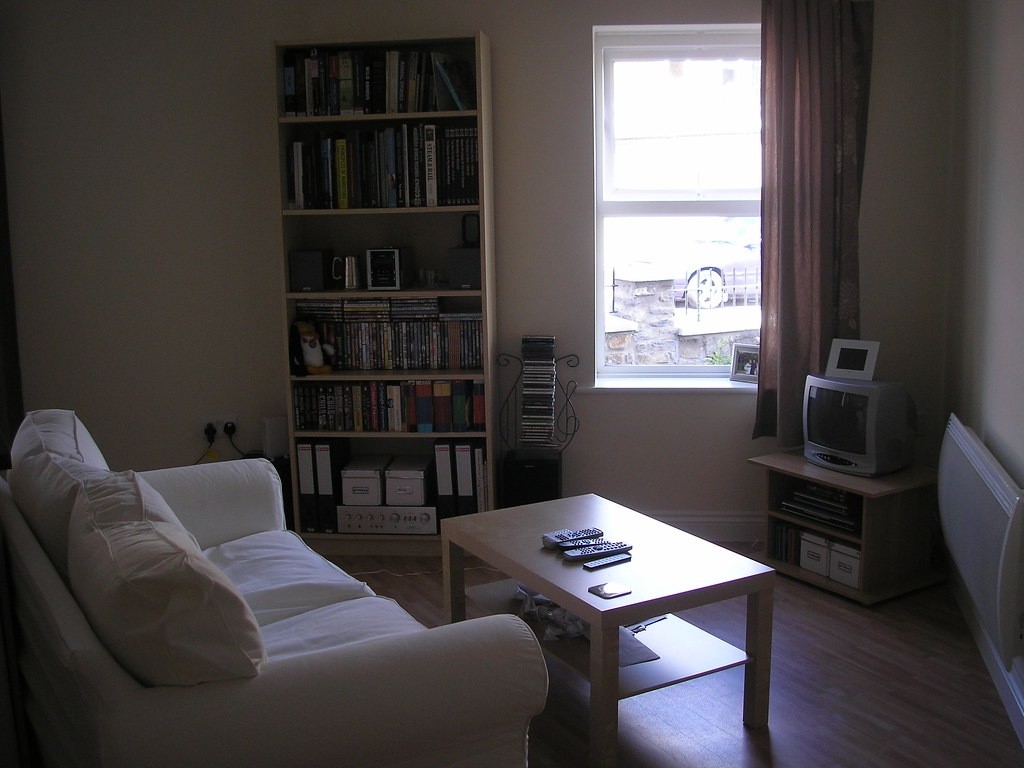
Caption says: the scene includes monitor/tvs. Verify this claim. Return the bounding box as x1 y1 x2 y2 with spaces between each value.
802 374 916 479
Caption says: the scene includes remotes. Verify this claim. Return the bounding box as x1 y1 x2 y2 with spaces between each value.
542 526 633 569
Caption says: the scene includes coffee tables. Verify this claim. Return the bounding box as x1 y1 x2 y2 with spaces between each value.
438 492 777 755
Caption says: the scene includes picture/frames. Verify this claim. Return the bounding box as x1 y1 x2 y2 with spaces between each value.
824 338 881 381
730 345 761 384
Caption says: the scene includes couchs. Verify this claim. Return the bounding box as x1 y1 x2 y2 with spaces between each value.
0 408 549 768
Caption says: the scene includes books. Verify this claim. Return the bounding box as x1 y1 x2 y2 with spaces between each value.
287 123 479 210
345 256 360 289
294 379 485 432
295 296 485 371
282 45 472 116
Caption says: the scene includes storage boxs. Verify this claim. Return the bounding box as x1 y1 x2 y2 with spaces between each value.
799 530 836 577
829 541 861 590
340 454 390 506
384 454 434 507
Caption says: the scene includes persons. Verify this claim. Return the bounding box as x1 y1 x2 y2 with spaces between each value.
744 359 759 375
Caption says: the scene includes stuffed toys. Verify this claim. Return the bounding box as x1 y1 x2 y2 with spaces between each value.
289 319 335 376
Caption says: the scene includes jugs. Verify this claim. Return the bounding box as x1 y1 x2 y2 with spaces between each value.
332 255 359 290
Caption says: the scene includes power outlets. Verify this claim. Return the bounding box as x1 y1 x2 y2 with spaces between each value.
199 415 239 440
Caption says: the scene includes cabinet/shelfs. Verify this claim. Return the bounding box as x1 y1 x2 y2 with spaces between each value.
746 449 940 606
269 30 499 559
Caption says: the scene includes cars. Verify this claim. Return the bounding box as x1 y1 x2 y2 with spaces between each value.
674 244 761 309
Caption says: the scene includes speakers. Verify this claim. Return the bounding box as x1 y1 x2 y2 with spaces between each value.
448 244 482 290
290 250 338 292
